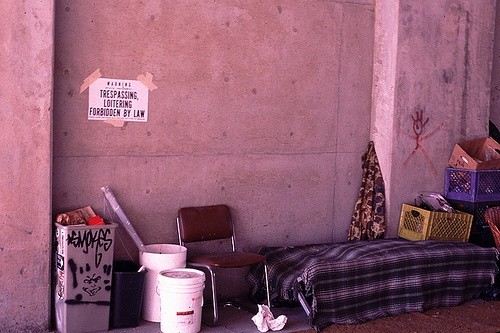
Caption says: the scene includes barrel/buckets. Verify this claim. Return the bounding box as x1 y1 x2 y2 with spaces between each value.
157 268 206 333
137 243 187 323
112 261 148 328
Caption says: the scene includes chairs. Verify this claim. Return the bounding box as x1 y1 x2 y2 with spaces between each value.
176 204 272 327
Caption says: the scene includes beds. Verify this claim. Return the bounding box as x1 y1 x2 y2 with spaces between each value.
245 237 500 333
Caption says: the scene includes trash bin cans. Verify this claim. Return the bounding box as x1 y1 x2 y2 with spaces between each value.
55 205 117 333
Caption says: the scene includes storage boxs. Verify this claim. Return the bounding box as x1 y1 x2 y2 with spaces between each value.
50 219 118 333
397 136 500 248
